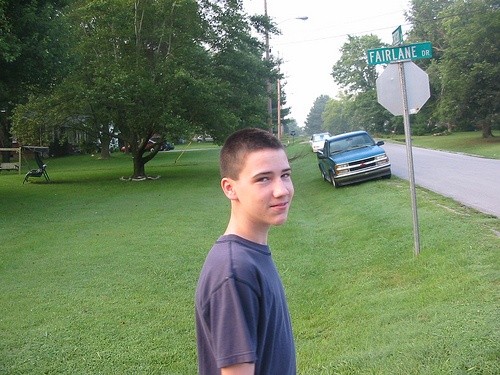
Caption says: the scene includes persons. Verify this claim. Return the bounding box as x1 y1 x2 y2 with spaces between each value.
195 128 296 375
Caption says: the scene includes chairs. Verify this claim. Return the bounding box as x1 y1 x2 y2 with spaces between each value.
23 152 50 185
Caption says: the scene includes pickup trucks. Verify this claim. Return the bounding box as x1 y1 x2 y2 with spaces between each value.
317 130 392 189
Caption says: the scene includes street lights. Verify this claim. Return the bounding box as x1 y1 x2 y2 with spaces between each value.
265 15 309 134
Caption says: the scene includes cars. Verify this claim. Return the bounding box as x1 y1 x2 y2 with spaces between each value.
309 132 334 153
197 134 216 144
120 136 176 153
